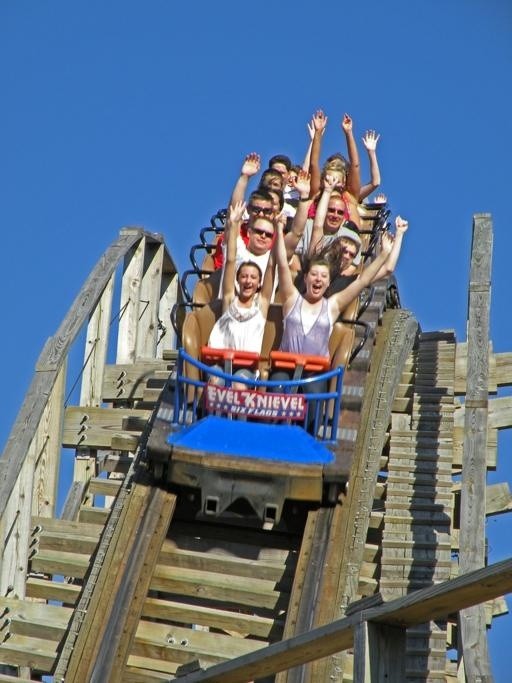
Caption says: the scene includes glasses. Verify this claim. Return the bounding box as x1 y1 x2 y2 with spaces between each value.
251 228 273 240
327 206 345 215
248 205 273 215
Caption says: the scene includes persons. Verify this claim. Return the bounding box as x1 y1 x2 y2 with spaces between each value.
202 110 408 436
